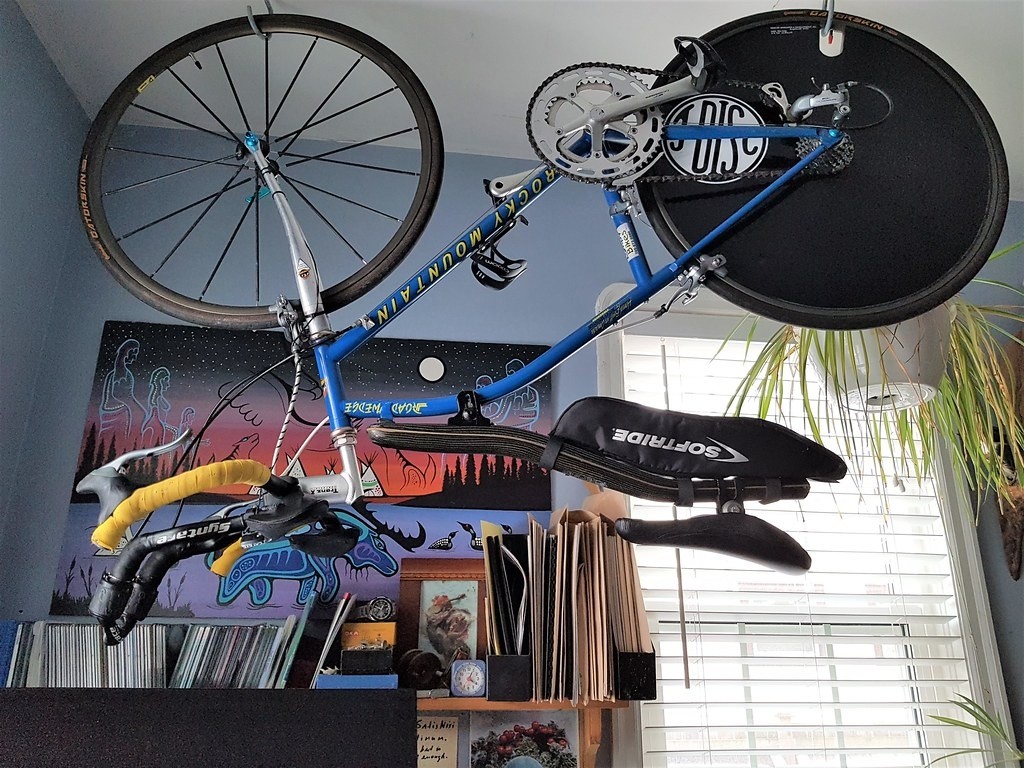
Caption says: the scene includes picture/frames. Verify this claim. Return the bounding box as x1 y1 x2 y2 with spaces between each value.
394 558 487 667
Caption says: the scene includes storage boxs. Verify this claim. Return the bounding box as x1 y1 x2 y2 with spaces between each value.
341 620 396 646
340 650 393 676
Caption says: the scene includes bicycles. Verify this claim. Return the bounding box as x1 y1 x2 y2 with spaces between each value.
77 11 1011 644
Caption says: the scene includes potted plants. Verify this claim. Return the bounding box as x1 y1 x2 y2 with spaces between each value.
711 240 1024 526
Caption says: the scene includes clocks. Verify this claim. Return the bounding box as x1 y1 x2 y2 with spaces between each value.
451 660 485 696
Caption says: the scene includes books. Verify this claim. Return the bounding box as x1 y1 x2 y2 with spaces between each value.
273 594 318 688
309 592 357 688
0 595 299 687
